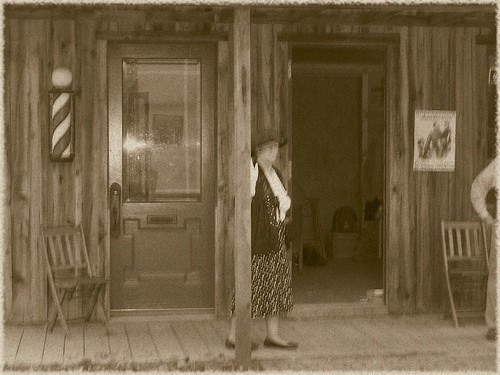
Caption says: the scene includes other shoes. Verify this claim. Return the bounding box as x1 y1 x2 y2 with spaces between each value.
263 338 298 348
226 337 259 351
486 328 498 339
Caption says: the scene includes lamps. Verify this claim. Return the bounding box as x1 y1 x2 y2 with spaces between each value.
50 68 75 163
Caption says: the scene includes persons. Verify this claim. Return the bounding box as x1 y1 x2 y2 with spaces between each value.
417 119 451 159
224 126 299 349
470 156 498 339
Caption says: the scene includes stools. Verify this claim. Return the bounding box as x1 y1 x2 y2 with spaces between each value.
299 196 325 263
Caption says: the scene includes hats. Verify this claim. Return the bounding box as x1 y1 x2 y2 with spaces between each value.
249 129 287 156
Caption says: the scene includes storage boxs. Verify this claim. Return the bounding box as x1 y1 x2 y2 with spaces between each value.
329 232 359 258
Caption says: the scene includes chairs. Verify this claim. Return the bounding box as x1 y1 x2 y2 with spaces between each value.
441 218 490 328
39 225 111 337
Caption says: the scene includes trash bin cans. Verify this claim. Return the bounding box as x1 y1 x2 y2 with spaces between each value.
324 206 358 261
